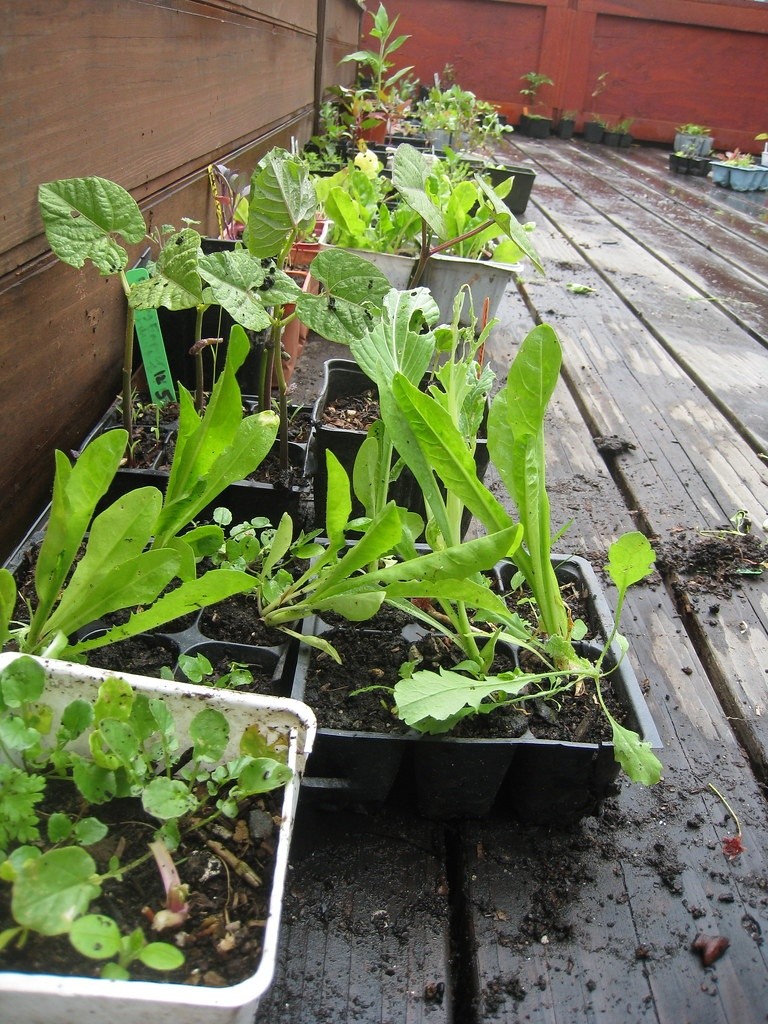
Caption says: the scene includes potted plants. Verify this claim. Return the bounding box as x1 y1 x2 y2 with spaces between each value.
672 123 715 156
669 144 714 176
604 120 621 147
145 0 536 399
293 281 667 823
0 322 528 699
30 141 392 573
556 109 578 139
311 283 501 541
519 71 554 138
582 71 610 142
709 151 768 192
0 569 316 1024
619 118 635 148
